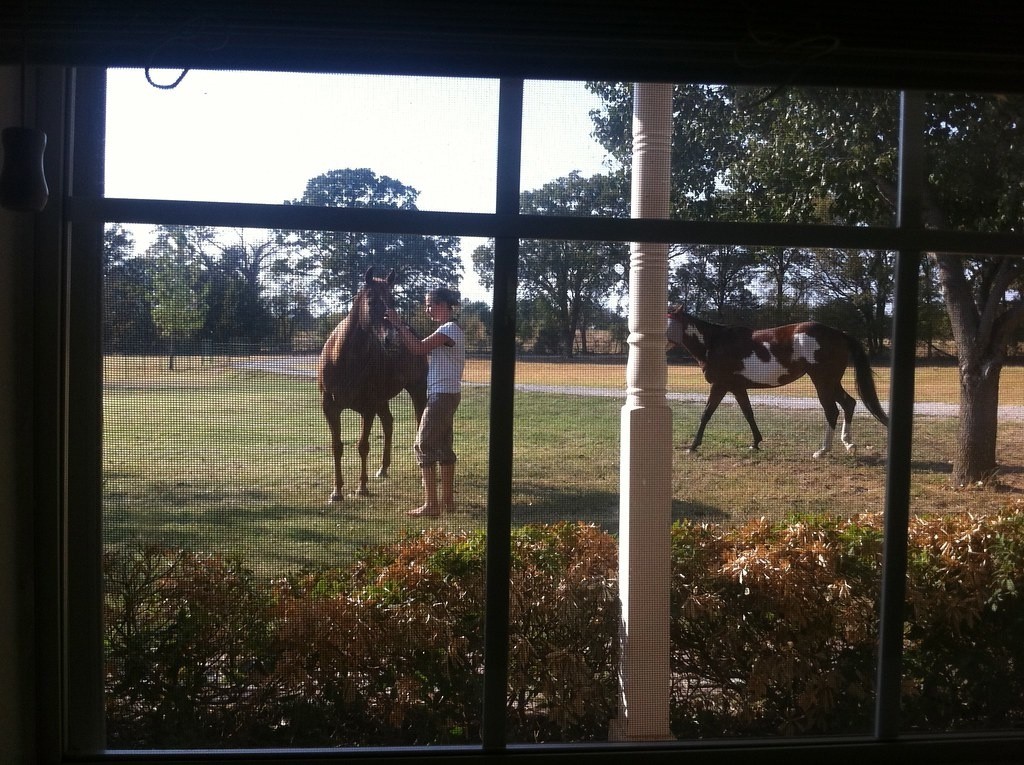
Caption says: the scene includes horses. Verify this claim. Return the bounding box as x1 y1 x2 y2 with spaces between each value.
315 264 431 506
663 302 889 461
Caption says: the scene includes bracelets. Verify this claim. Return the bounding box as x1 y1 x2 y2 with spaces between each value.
394 322 407 332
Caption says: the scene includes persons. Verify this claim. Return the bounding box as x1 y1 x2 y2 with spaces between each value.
382 287 467 518
